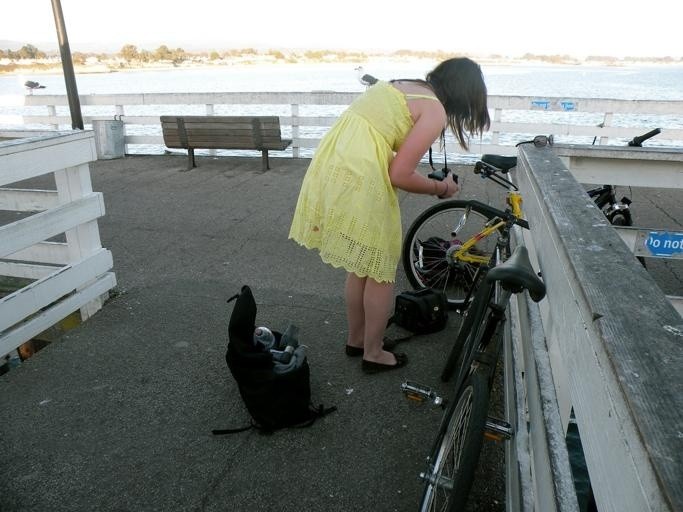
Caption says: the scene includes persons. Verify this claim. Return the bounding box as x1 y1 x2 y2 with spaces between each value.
287 55 491 375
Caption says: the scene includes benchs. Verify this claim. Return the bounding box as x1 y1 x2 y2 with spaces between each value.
155 109 294 173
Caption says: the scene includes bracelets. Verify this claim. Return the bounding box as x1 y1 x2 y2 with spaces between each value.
431 179 438 196
438 181 448 197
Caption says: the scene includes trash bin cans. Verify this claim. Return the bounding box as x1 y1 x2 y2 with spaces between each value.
92 120 126 161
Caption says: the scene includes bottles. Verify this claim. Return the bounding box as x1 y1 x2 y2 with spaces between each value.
255 325 273 348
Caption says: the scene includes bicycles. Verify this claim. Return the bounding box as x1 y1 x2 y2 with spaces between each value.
393 126 660 312
400 200 544 512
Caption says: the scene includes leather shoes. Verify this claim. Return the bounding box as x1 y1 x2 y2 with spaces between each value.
346 339 395 357
362 351 407 375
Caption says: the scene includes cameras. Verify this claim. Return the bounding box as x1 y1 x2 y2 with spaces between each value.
428 167 458 199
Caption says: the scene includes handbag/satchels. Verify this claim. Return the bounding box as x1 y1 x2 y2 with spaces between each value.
225 285 310 431
394 287 448 335
